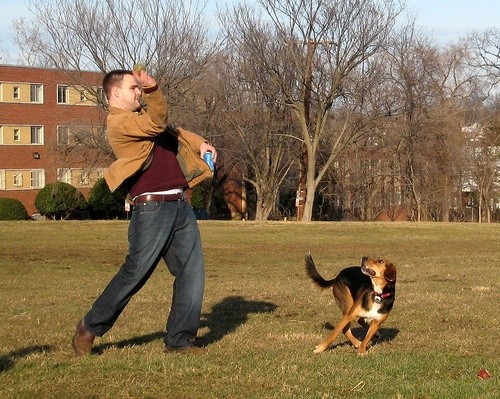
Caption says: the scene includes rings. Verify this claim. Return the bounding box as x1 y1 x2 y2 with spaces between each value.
212 147 216 150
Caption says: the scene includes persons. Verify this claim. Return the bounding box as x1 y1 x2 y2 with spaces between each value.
72 69 218 356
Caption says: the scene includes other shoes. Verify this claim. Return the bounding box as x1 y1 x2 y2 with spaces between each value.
72 319 95 357
164 345 209 356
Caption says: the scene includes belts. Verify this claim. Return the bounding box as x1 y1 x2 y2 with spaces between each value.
131 192 185 204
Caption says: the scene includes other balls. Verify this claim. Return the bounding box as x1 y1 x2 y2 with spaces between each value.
135 64 144 76
478 370 492 381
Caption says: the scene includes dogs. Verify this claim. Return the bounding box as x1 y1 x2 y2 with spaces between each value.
304 251 397 357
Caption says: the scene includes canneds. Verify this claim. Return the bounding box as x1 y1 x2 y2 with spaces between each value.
203 151 214 177
